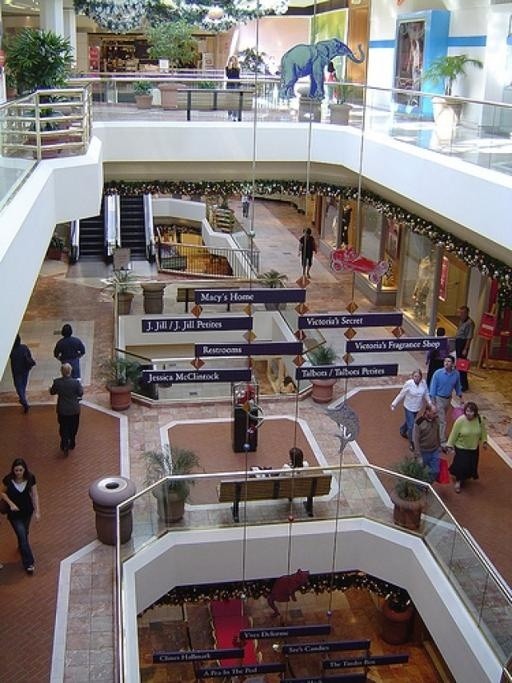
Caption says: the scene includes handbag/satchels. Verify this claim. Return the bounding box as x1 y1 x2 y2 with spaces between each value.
436 458 450 484
457 354 471 371
1 500 10 515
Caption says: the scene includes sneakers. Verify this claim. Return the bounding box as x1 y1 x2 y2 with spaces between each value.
409 441 415 451
400 431 408 439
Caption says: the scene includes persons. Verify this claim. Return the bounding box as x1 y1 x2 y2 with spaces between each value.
0 456 40 572
48 362 84 459
240 194 252 219
270 447 310 479
9 333 37 414
326 61 338 99
388 304 488 495
223 53 242 122
51 322 86 382
296 228 318 280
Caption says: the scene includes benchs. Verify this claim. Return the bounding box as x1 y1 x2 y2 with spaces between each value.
217 472 332 523
176 89 253 122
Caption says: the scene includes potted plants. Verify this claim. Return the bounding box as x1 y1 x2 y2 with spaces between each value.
47 232 64 259
388 456 434 529
260 269 288 311
138 443 206 522
97 356 144 410
328 75 355 125
146 18 199 111
2 25 77 158
105 269 141 315
306 345 340 403
423 55 483 140
132 79 153 109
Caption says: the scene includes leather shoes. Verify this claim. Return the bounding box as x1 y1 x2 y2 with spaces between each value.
26 565 34 576
455 480 461 493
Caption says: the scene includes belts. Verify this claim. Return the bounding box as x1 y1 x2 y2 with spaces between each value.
437 394 452 399
